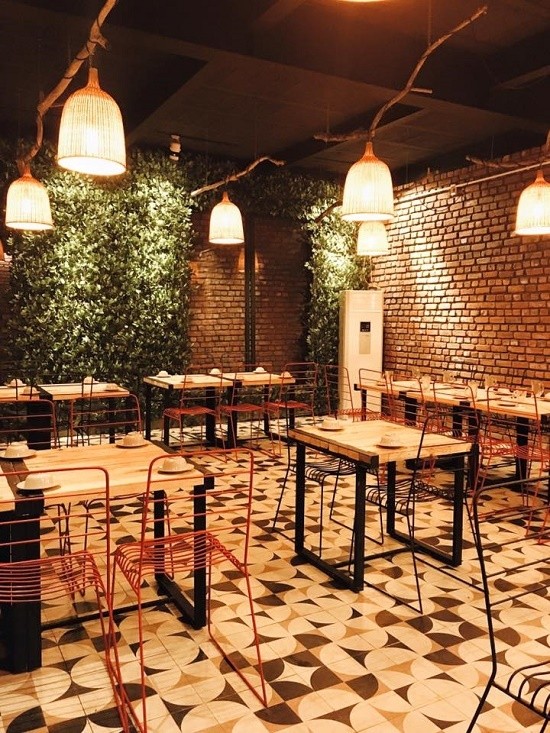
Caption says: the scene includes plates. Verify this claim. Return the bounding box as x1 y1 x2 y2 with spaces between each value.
19 392 41 395
16 480 61 490
540 397 550 401
498 403 518 406
453 385 468 388
7 384 27 387
117 440 150 447
84 382 100 385
495 392 513 395
375 383 396 386
104 389 120 391
158 464 195 472
0 450 36 458
156 371 293 383
376 442 407 448
316 424 344 430
410 389 421 392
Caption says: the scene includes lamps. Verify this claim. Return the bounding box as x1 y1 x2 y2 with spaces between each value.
54 52 126 177
513 159 550 235
208 175 245 245
357 221 389 256
340 130 396 221
4 158 54 231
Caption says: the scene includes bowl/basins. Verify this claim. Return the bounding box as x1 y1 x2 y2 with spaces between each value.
162 456 187 470
159 366 291 380
122 432 145 445
10 379 23 385
24 474 55 488
23 386 37 394
321 418 402 446
106 384 118 388
5 445 31 456
83 377 94 383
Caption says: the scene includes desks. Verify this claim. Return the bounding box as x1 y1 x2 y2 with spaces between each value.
287 420 482 594
0 382 130 451
357 380 550 493
0 440 214 675
142 372 295 451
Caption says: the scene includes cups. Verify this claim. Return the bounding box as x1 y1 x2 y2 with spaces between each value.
385 368 545 410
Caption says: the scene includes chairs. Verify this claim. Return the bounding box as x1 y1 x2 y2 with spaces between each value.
0 358 550 733
